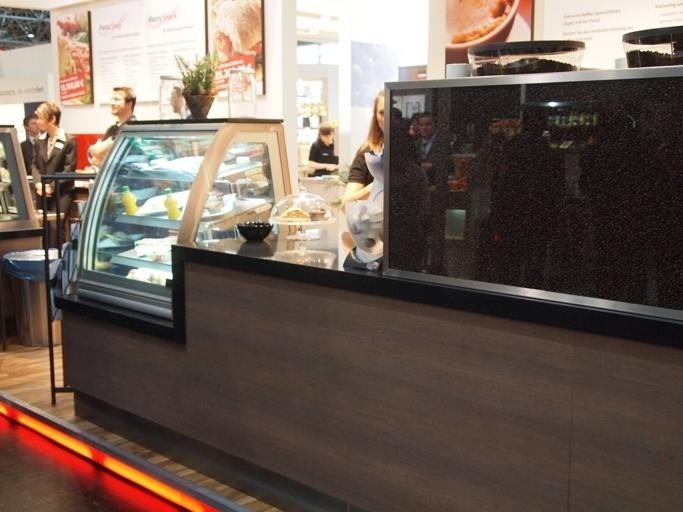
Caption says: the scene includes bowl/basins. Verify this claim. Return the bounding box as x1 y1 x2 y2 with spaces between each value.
237 221 274 242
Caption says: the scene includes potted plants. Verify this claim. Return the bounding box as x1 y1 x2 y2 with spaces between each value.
173 49 219 119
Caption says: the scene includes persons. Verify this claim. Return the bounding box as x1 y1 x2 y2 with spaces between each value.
86 87 136 170
18 114 43 177
461 102 645 298
29 101 77 248
392 108 455 276
166 83 201 119
305 123 341 178
338 89 387 231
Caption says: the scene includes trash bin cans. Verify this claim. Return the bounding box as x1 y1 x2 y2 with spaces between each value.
2 247 63 346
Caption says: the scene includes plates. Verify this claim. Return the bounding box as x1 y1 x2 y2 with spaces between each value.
139 190 191 216
200 193 237 218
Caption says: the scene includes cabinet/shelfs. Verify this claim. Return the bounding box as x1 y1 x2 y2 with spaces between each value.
382 66 683 321
0 125 41 231
54 120 293 322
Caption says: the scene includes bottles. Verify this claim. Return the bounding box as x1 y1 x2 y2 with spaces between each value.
121 185 139 216
164 188 181 220
